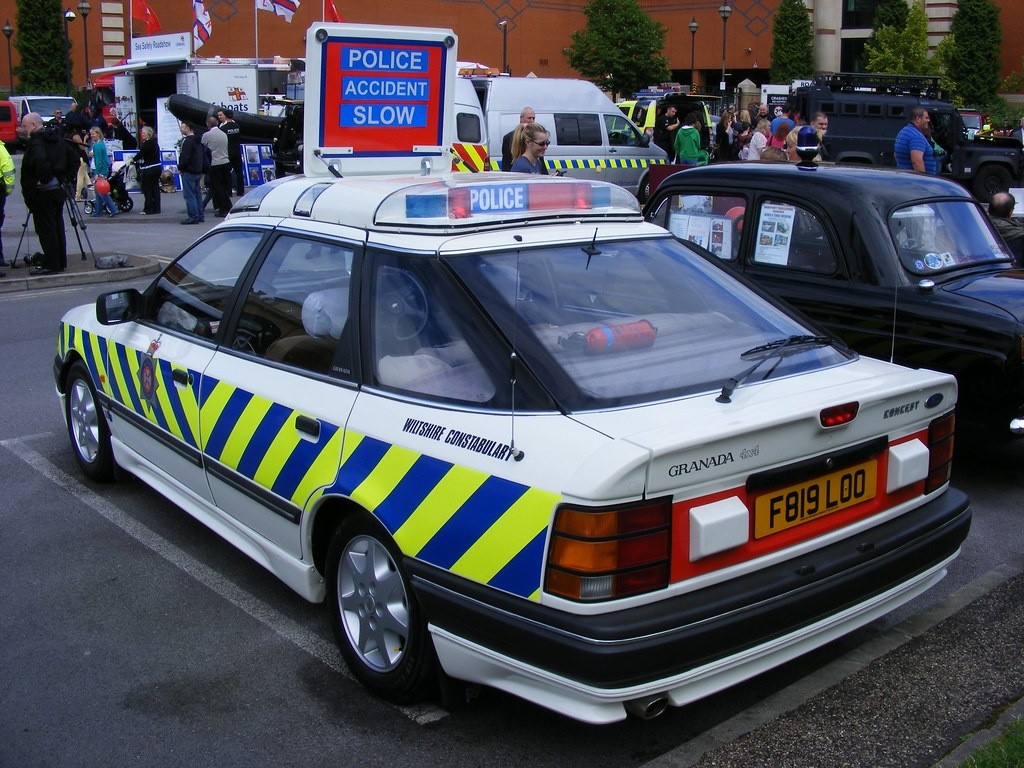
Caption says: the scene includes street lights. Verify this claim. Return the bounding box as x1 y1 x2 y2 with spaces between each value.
688 16 700 92
63 6 79 97
718 0 732 114
77 0 92 88
1 18 14 96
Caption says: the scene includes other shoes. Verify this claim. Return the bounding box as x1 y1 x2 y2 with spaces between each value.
30 266 64 276
90 213 97 217
181 218 198 224
0 272 6 278
214 213 225 217
0 259 9 266
199 219 205 222
140 211 146 215
109 210 119 217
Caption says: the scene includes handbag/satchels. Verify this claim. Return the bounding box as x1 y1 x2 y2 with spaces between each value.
125 164 143 192
201 141 212 175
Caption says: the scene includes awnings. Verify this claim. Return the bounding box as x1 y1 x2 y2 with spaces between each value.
91 59 188 74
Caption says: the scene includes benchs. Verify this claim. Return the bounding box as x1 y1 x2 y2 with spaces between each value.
378 309 726 388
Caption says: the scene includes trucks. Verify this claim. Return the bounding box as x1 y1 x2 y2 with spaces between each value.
91 32 488 174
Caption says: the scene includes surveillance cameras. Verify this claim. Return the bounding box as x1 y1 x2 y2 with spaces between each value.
65 12 76 21
500 21 506 26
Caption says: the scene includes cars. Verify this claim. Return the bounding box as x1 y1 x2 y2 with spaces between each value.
637 162 1024 455
52 173 974 726
959 110 988 132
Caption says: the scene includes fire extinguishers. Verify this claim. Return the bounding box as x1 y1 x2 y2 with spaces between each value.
557 318 659 356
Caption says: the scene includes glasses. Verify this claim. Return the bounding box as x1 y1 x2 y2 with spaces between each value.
523 138 550 147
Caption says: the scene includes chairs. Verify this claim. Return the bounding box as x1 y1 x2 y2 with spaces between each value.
264 286 352 374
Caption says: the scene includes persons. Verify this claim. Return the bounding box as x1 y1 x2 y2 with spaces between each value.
178 121 205 224
264 168 275 182
50 103 162 218
987 191 1024 253
894 106 936 175
502 107 548 172
1011 117 1024 151
0 140 16 277
510 123 550 174
202 108 244 216
716 103 829 162
20 112 67 276
653 104 709 166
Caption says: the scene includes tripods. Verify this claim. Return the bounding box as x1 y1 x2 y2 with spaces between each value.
11 183 98 267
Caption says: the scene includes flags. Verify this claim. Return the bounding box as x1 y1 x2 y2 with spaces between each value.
324 0 343 22
132 0 160 36
193 0 212 50
257 0 301 23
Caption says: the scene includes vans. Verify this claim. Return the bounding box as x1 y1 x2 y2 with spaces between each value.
608 86 714 159
0 95 78 153
472 78 670 204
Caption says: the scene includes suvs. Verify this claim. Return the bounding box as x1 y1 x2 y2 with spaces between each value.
792 71 1024 203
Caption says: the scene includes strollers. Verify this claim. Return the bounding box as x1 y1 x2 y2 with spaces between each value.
81 159 137 215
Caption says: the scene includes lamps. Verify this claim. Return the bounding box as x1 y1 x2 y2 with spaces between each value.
744 48 752 53
